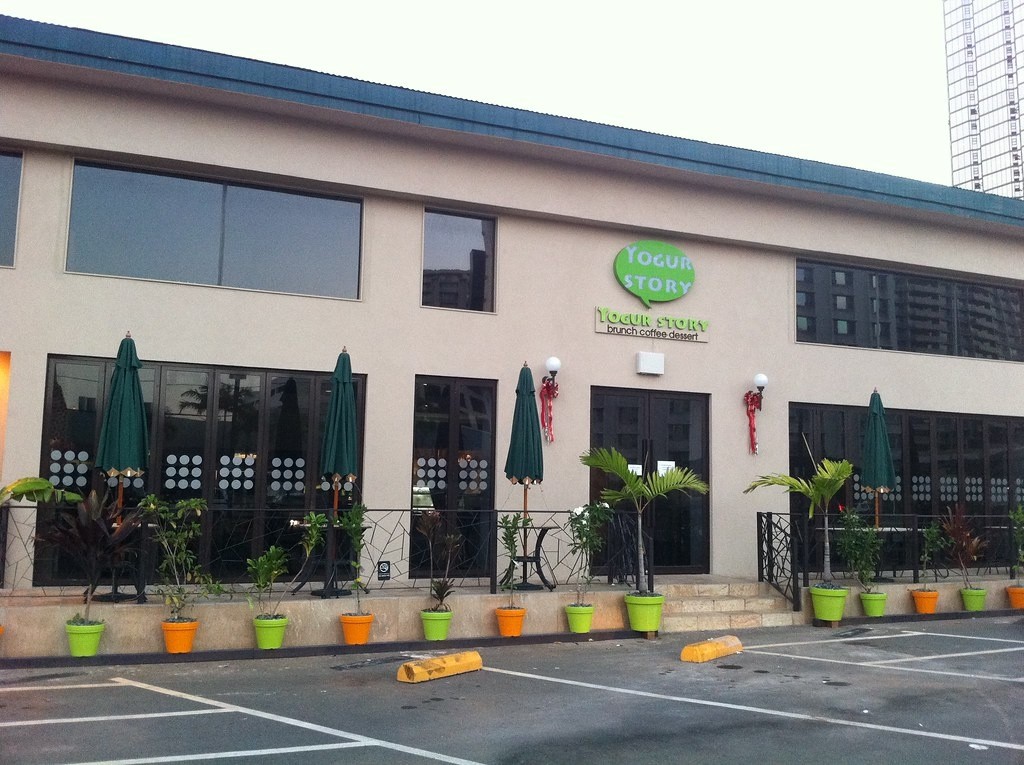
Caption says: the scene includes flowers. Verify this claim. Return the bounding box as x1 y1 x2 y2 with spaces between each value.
567 501 614 606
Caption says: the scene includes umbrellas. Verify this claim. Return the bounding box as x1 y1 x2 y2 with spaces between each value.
503 361 544 590
309 345 358 595
95 330 147 602
861 387 897 582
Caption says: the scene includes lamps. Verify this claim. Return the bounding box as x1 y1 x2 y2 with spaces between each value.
542 357 560 398
745 375 768 411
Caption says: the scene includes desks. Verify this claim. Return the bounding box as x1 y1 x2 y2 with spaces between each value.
87 524 158 603
986 526 1019 578
499 525 561 592
290 522 372 598
817 528 940 578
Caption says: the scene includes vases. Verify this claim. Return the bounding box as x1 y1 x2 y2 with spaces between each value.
565 603 593 634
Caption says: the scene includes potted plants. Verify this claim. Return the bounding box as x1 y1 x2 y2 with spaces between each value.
489 514 533 637
937 502 988 610
742 458 853 622
912 520 956 614
416 510 465 642
578 448 710 633
50 488 152 657
245 513 330 650
134 492 225 654
835 506 886 616
1007 504 1024 610
330 503 375 645
